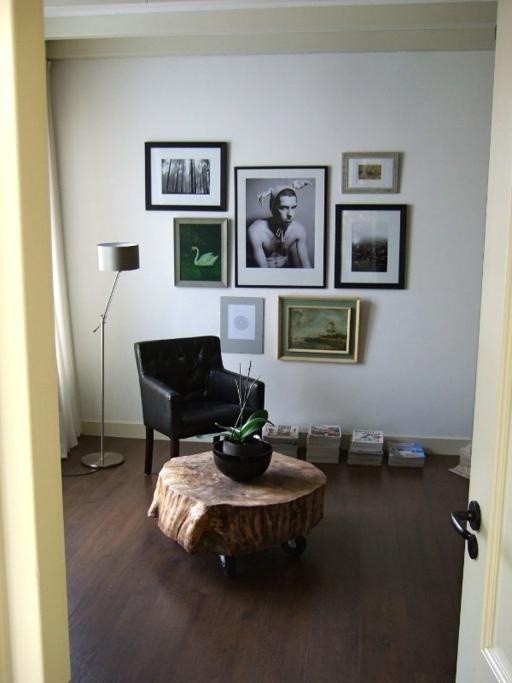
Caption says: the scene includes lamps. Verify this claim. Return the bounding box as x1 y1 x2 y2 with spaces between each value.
79 242 140 469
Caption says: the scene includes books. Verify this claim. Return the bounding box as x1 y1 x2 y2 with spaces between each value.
255 422 299 458
305 424 342 464
346 430 383 467
449 442 471 478
386 442 426 468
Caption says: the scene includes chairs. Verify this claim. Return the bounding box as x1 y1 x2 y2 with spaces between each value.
132 334 265 474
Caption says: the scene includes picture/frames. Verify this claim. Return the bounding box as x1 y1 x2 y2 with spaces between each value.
233 166 328 289
333 203 408 288
172 217 229 289
219 296 265 354
145 141 228 212
341 151 401 195
276 294 362 365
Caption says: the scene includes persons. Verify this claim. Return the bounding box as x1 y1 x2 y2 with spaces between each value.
248 181 311 268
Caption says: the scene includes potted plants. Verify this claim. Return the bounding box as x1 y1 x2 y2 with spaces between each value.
214 409 275 456
